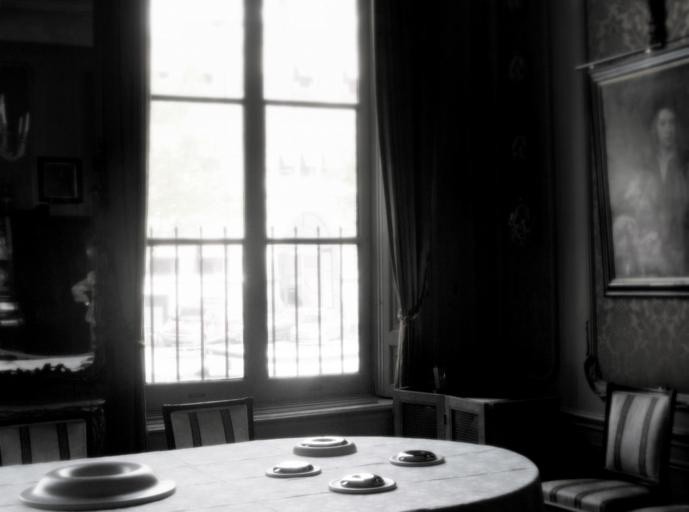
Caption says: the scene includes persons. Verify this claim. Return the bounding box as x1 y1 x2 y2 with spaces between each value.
620 104 689 277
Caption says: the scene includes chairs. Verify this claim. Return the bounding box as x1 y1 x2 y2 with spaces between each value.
538 379 677 512
1 396 255 468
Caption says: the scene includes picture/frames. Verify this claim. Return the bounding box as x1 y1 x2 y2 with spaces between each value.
35 155 84 204
581 42 689 299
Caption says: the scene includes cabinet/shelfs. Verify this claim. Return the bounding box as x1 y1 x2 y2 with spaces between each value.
390 387 527 446
